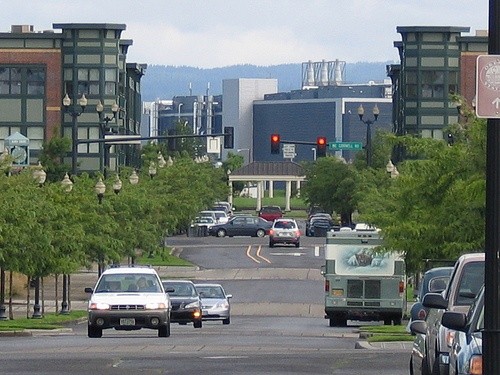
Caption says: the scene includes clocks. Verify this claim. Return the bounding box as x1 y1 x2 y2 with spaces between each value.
12 147 27 164
3 146 10 156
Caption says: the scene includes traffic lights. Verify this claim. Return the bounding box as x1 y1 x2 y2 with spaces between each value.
318 137 327 158
271 134 280 154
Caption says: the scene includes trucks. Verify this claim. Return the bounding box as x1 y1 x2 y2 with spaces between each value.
319 221 409 327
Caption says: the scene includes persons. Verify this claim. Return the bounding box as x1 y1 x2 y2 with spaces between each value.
136 277 149 291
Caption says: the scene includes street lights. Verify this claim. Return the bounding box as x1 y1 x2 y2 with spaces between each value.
358 104 381 167
94 176 105 277
95 101 121 175
29 161 46 318
146 152 209 262
130 169 136 267
62 91 87 176
58 173 69 315
111 172 120 268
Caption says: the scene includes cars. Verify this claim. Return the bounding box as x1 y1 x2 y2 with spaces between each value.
158 279 204 326
193 283 234 324
189 201 235 236
409 260 455 332
207 215 274 238
258 205 282 221
305 205 340 238
83 263 170 337
411 253 486 375
443 284 484 375
269 218 300 247
